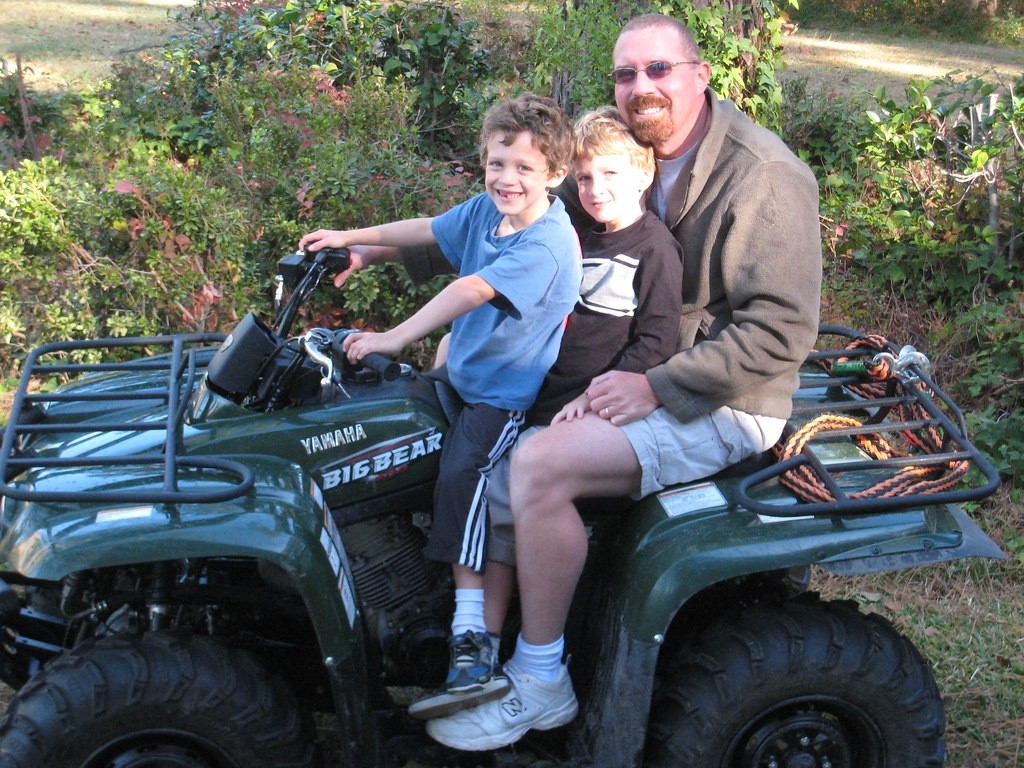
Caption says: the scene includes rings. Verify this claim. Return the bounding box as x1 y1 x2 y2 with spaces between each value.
604 406 613 419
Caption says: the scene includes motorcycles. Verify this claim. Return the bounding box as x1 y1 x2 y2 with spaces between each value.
2 244 1010 767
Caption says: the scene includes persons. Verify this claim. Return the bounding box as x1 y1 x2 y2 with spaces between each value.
298 95 583 721
332 14 824 751
482 104 685 668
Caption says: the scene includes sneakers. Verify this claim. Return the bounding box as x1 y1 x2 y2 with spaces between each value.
425 653 579 751
407 660 509 720
445 630 494 694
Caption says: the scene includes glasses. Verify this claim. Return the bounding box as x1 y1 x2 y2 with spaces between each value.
608 60 699 84
